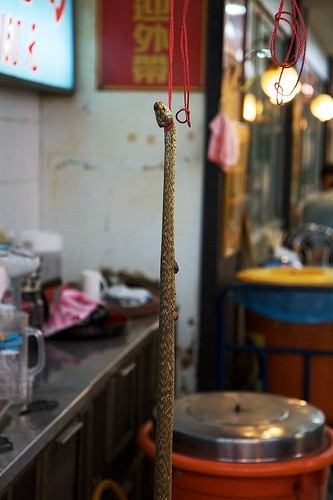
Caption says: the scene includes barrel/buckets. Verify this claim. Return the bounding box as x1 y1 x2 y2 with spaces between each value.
141 391 333 500
235 266 333 422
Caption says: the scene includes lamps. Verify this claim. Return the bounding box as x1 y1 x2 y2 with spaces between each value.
228 49 301 124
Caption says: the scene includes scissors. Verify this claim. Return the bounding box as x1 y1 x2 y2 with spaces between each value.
18 399 58 416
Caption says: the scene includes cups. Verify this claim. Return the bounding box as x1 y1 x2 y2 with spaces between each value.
81 270 107 301
0 311 45 405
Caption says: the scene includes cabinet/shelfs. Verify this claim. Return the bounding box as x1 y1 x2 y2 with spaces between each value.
0 302 181 500
